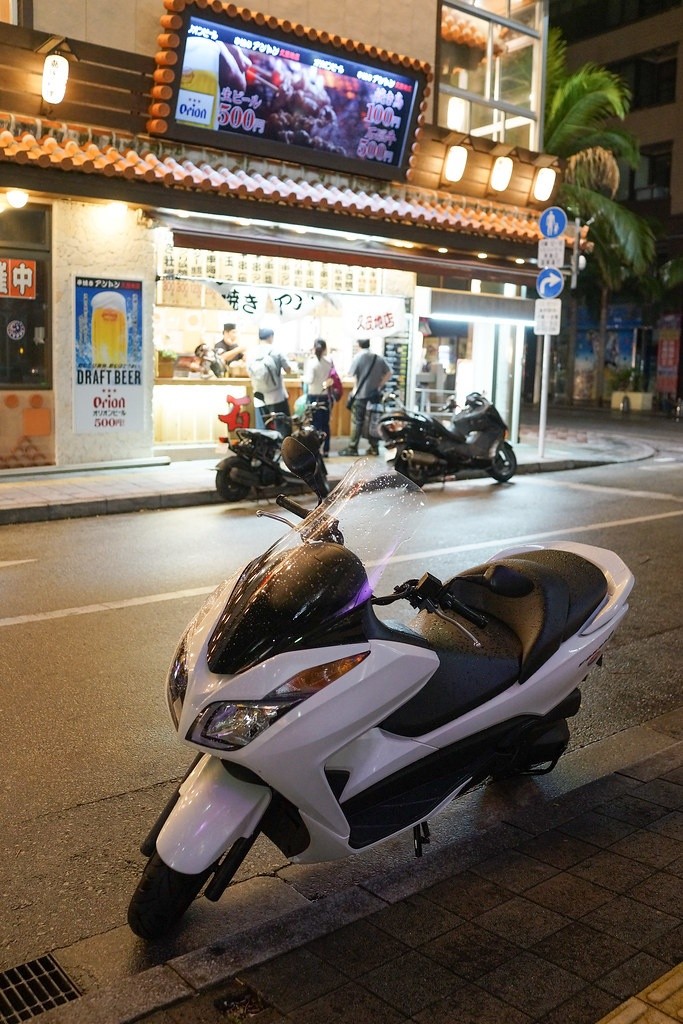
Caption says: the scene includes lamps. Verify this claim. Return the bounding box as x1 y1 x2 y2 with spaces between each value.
484 143 522 196
526 155 561 205
36 35 82 120
437 132 476 189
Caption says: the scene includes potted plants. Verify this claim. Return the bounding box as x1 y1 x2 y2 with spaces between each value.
611 366 652 411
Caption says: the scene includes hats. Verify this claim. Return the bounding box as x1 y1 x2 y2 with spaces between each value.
224 323 236 331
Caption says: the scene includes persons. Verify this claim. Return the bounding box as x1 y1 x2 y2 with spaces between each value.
337 337 392 456
303 338 344 458
247 327 292 439
214 323 246 363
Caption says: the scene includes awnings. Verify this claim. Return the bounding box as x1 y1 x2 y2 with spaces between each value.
146 206 550 287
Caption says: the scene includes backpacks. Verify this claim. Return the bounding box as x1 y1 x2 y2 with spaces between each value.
249 348 281 393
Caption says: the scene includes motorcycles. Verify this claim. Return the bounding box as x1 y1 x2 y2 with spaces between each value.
124 437 636 944
381 390 519 492
210 379 335 500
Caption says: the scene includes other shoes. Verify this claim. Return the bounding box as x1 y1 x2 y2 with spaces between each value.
339 444 358 456
323 450 328 457
366 445 379 456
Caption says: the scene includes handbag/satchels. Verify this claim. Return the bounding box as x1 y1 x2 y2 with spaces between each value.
329 360 343 402
345 392 355 410
